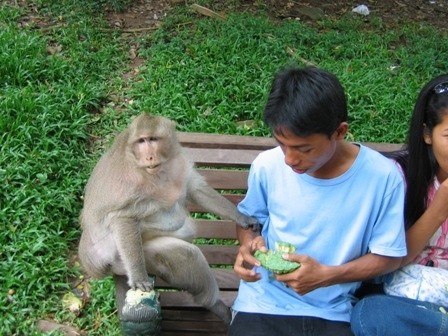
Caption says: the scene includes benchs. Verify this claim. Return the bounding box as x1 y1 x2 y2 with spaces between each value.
116 133 409 336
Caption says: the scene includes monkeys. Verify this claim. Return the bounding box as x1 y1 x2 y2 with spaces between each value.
79 113 259 324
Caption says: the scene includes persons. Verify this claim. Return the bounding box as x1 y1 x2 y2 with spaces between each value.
351 73 448 336
222 66 408 335
